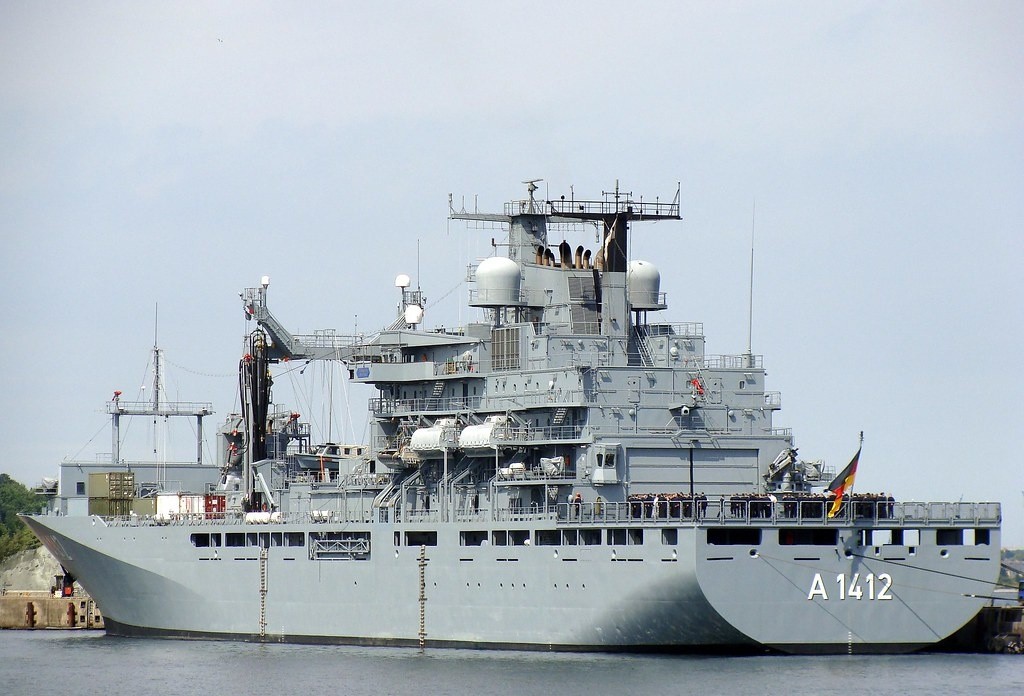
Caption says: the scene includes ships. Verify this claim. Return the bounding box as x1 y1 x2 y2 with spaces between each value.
18 179 1024 659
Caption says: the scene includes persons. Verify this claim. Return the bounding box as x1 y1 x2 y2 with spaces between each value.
718 494 727 519
623 490 709 519
728 490 899 521
574 492 583 519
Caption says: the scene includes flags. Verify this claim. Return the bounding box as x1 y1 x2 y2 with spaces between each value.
822 447 862 518
